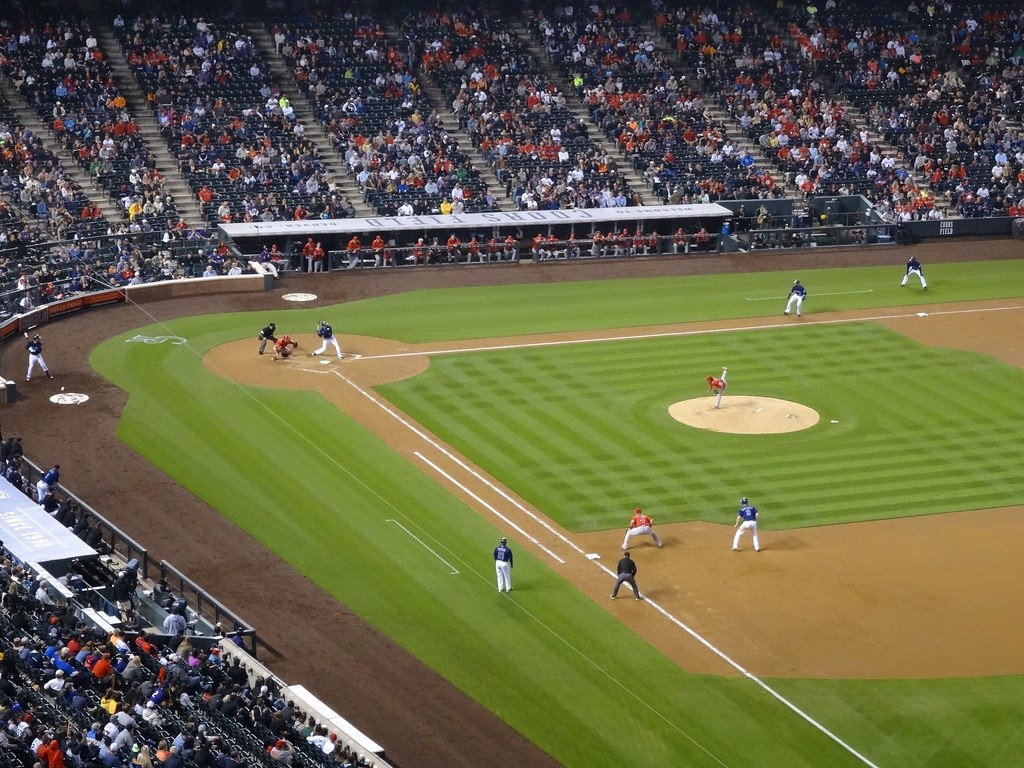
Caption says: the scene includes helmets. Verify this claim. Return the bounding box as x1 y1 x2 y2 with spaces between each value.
705 375 713 381
635 508 641 514
269 323 275 326
321 321 328 328
284 335 292 342
740 497 748 505
501 538 507 544
908 256 916 262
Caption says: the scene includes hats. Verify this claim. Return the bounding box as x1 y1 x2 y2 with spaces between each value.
10 570 336 750
793 279 800 283
263 228 709 255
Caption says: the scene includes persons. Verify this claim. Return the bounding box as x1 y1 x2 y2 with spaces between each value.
446 235 463 264
466 238 483 263
621 508 662 550
0 435 381 768
412 239 432 265
900 257 927 290
784 280 808 317
704 366 728 409
311 321 343 360
383 240 394 266
347 236 360 268
258 323 278 354
731 497 760 551
504 236 518 261
610 552 641 600
493 537 514 593
0 0 1024 320
271 336 297 360
371 235 386 266
488 239 501 261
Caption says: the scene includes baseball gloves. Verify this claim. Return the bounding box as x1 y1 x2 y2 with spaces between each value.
293 342 298 348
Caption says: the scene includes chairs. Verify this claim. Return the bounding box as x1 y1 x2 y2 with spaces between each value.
0 545 375 768
0 0 1023 325
